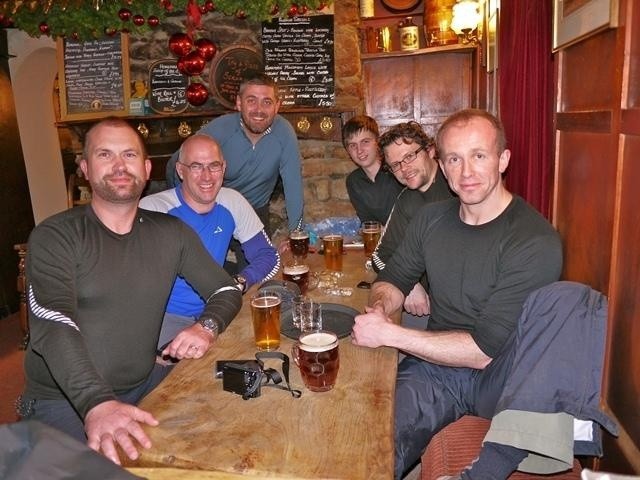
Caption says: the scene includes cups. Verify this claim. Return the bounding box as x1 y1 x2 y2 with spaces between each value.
289 230 310 264
291 295 313 329
363 221 381 257
291 330 340 392
300 301 322 335
322 235 343 273
250 289 281 352
282 262 309 300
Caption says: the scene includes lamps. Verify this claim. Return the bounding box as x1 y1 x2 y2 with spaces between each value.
449 1 484 69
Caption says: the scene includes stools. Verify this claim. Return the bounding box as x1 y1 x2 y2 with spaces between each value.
421 413 582 480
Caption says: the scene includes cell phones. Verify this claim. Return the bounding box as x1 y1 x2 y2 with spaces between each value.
215 359 265 378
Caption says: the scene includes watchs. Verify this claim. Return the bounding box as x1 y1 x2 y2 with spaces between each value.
196 318 220 340
233 274 248 292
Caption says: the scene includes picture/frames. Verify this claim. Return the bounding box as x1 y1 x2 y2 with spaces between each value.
210 47 269 112
147 59 191 115
55 33 132 121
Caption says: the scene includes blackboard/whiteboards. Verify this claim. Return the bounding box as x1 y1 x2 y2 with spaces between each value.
260 14 336 109
57 32 125 114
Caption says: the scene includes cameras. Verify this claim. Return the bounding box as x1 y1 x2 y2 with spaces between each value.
221 362 261 399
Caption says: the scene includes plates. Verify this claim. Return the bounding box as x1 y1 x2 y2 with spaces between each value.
280 303 361 341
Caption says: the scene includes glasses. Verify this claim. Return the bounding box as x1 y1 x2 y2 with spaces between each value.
387 145 423 174
177 160 223 172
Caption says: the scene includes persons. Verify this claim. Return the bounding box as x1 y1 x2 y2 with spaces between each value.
350 108 619 480
372 121 449 317
16 115 243 467
341 114 406 226
139 133 280 365
166 72 305 236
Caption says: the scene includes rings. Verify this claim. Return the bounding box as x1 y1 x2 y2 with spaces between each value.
189 345 199 350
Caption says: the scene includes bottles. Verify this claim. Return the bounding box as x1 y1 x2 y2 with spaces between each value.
399 17 419 51
